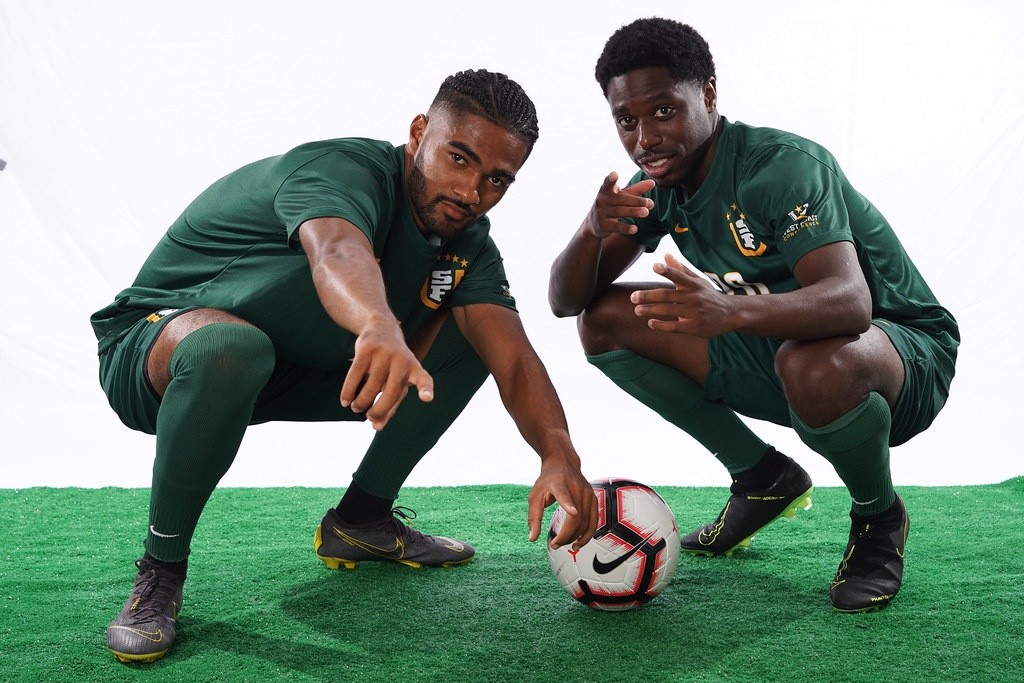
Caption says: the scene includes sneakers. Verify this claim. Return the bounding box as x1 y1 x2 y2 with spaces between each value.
312 508 476 569
677 456 814 558
829 492 910 613
107 567 184 664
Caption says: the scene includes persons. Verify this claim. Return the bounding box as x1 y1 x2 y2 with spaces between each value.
90 68 599 664
547 15 961 611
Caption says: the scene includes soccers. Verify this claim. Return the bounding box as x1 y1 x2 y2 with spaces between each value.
547 477 682 612
